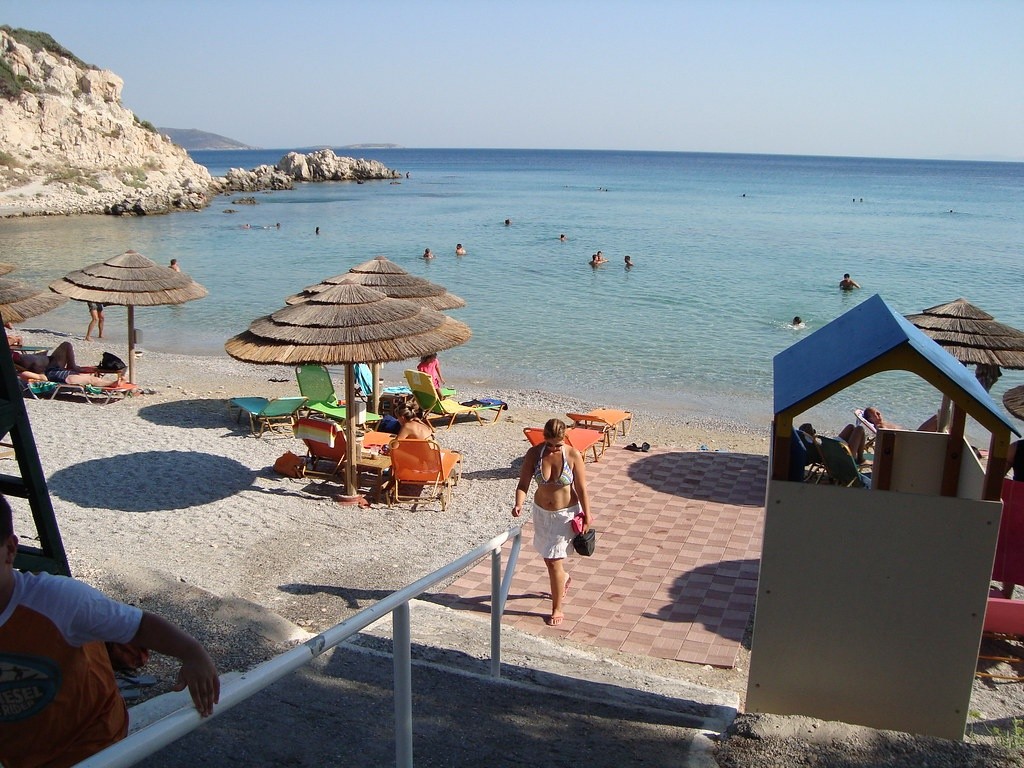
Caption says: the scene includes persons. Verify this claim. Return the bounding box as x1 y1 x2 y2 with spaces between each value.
511 417 591 625
424 248 433 258
862 406 938 432
85 302 105 340
455 244 467 255
0 493 220 767
799 422 873 466
8 334 127 388
793 317 801 325
840 274 861 291
591 251 608 265
169 258 180 271
624 255 634 266
416 352 447 401
388 401 442 470
1003 439 1024 482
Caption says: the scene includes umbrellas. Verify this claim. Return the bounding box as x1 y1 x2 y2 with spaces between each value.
901 296 1024 432
0 262 68 326
225 279 472 495
286 256 465 415
47 249 207 384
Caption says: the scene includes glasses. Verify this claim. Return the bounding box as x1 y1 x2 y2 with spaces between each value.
545 440 564 448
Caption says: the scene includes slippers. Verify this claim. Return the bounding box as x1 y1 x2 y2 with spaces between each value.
548 612 564 625
552 577 572 600
642 442 650 452
696 445 708 450
624 443 641 451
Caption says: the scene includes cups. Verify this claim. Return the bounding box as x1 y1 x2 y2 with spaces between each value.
371 446 378 460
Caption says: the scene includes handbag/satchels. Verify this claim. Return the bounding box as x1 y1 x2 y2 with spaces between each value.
273 451 304 479
97 352 125 371
569 512 596 556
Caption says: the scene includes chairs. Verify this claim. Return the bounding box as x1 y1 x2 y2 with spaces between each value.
523 409 633 464
10 343 136 406
227 364 509 513
797 410 879 488
